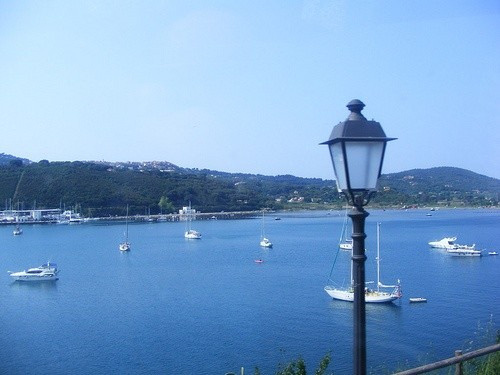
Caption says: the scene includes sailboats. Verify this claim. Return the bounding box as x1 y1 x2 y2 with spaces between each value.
255 257 263 262
323 220 402 303
184 202 202 240
119 204 132 252
0 199 101 225
259 209 274 248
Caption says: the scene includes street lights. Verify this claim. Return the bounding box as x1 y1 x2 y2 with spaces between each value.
317 97 398 375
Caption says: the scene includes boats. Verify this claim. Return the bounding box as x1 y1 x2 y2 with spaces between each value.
339 220 354 250
425 213 432 217
489 251 500 256
275 216 281 221
12 226 23 235
428 207 439 211
428 237 476 249
409 297 428 303
212 216 217 219
447 244 483 258
327 212 331 217
8 260 61 282
